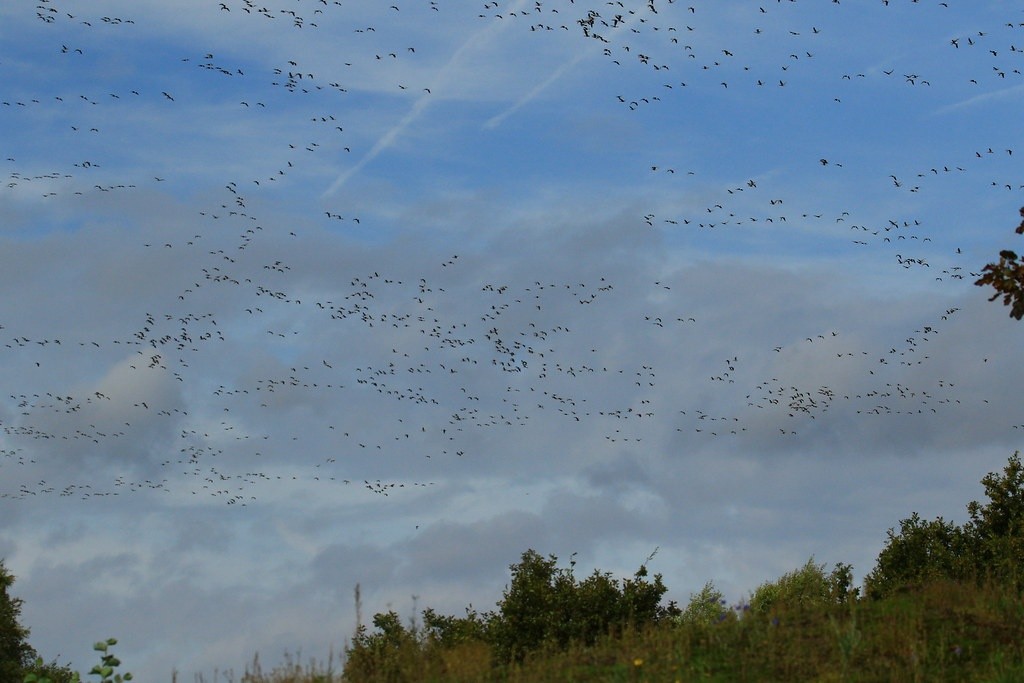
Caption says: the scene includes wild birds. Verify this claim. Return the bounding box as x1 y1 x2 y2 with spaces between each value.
0 0 1024 511
